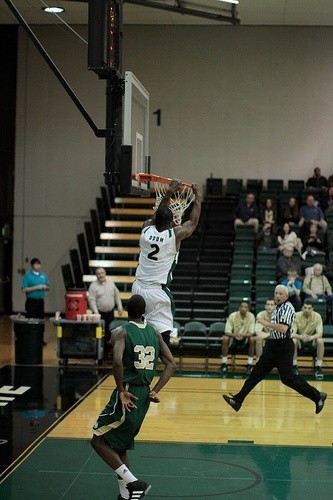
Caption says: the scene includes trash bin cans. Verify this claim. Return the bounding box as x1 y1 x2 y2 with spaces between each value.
11 318 46 364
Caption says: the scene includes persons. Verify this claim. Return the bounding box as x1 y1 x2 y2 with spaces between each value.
292 300 324 380
255 298 276 363
221 300 255 374
131 178 201 344
91 294 177 500
230 167 333 312
21 258 50 345
88 267 123 360
222 285 327 414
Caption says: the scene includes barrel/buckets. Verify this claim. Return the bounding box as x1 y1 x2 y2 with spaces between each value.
65 288 87 320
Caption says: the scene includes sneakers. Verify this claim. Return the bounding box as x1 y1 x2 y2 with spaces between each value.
117 494 126 500
315 366 323 377
294 364 298 375
125 480 151 500
315 391 327 414
219 362 228 373
223 393 241 412
246 363 253 374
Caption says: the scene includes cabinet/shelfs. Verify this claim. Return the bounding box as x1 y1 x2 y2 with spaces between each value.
50 318 105 361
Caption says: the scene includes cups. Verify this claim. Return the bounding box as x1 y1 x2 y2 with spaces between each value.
76 309 101 322
55 311 60 320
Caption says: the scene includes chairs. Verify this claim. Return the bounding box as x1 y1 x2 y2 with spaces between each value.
110 176 329 372
